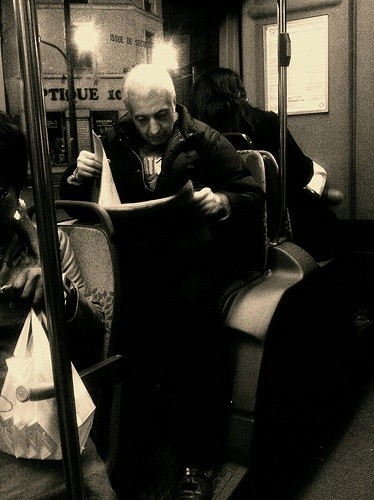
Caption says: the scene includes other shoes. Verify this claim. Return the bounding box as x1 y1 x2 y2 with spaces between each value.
175 463 214 500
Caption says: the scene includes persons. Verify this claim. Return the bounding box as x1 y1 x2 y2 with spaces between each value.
59 62 266 500
0 111 116 500
189 66 374 335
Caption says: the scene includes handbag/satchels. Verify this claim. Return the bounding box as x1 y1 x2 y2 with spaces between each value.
0 306 96 460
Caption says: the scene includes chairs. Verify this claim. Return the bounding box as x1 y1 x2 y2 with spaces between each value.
220 132 319 467
258 150 337 289
26 201 124 497
